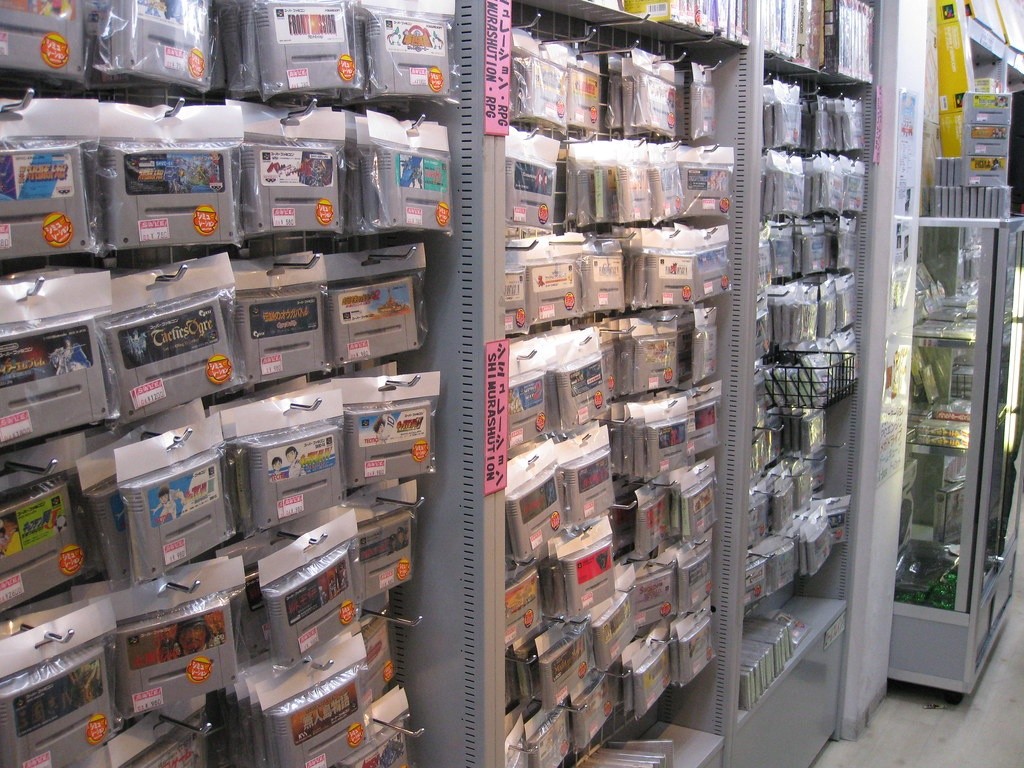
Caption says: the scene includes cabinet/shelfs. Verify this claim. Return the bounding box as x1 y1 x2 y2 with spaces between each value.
0 1 500 768
884 214 1024 712
707 1 922 768
489 0 761 768
